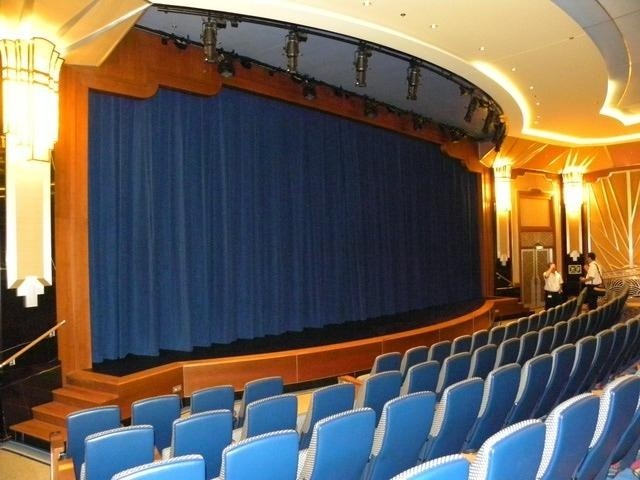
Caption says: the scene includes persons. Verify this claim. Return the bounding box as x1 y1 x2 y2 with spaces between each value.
576 266 580 272
579 252 603 310
543 263 564 310
571 266 575 273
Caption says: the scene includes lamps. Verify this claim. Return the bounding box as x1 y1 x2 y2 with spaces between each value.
464 96 477 123
301 80 318 99
493 163 512 212
491 122 503 143
363 101 377 118
199 15 219 65
407 59 421 100
561 170 584 214
481 110 495 132
0 38 65 161
283 32 300 71
413 116 425 130
218 50 234 79
450 129 463 144
352 45 370 88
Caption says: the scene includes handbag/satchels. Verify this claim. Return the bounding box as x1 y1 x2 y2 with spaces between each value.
590 284 607 296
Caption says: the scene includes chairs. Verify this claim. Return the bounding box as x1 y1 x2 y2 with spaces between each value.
66 285 640 480
